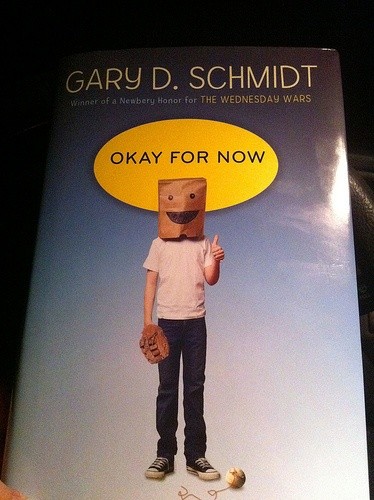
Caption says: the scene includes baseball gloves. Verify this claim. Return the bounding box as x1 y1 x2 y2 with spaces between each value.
139 325 168 364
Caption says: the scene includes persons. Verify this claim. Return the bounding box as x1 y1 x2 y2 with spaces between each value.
141 177 223 483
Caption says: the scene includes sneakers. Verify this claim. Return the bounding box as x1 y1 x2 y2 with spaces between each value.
186 458 220 480
145 456 173 479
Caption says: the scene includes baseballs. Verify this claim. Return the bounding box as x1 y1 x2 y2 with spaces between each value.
227 467 245 488
